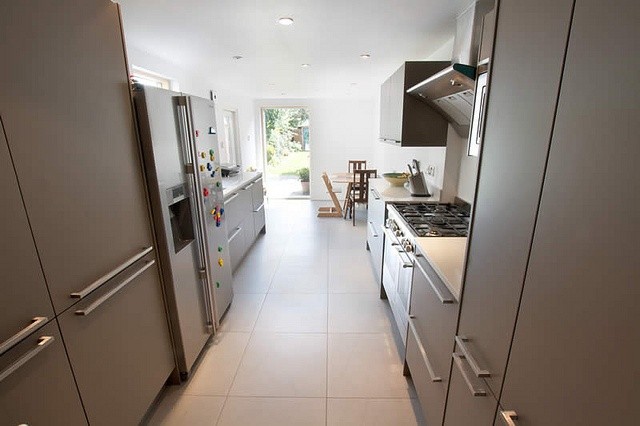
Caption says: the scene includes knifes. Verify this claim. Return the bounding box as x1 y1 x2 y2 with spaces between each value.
413 160 421 174
407 164 413 176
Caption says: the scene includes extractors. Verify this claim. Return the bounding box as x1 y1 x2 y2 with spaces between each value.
405 0 495 139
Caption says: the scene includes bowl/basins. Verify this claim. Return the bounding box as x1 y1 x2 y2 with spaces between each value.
381 172 411 188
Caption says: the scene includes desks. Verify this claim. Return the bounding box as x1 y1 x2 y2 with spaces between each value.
330 172 385 220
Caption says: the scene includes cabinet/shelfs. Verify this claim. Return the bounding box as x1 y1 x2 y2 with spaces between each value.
252 173 266 238
378 60 452 148
0 1 182 426
365 181 385 299
401 245 458 425
441 1 639 426
466 9 495 157
224 182 256 275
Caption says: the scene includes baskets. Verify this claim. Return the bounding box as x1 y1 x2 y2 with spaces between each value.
384 173 409 186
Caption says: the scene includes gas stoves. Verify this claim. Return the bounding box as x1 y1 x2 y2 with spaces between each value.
390 201 471 239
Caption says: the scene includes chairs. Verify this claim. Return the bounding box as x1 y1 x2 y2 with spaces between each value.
348 169 377 227
343 160 367 219
317 170 343 217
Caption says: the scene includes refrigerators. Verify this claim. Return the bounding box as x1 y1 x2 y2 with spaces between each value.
131 82 235 382
